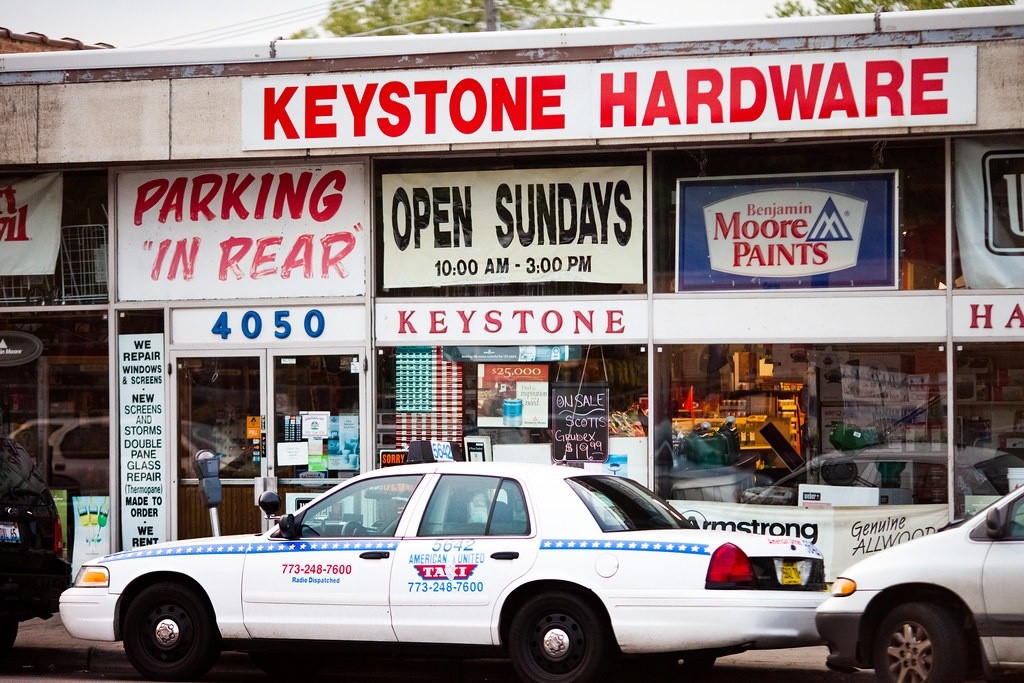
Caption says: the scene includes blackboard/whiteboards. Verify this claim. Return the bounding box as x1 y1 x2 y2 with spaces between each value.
552 382 609 462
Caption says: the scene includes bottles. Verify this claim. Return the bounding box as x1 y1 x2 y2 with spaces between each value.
96 497 110 527
89 497 98 526
999 432 1006 448
747 423 755 446
873 461 907 489
738 427 747 446
76 497 89 526
494 382 499 396
701 422 711 431
976 380 996 401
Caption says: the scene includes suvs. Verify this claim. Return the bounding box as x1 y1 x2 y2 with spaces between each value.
0 435 74 667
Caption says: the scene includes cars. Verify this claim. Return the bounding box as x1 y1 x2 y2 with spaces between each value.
738 441 1024 518
815 483 1024 683
57 443 829 683
5 413 256 496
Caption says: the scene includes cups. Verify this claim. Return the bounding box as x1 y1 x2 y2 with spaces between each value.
342 450 350 463
1015 423 1024 432
503 398 522 426
349 454 358 469
722 399 746 406
722 411 746 417
1007 468 1024 515
602 455 627 478
331 430 338 439
